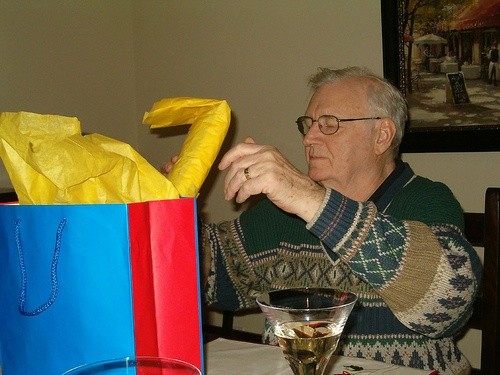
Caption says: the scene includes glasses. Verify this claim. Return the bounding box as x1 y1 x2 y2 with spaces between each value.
294 114 381 136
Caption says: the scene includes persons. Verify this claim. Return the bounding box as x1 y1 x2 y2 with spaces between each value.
486 41 500 86
424 47 434 71
156 68 483 375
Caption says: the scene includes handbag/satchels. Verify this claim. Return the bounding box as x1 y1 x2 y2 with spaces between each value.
0 191 204 375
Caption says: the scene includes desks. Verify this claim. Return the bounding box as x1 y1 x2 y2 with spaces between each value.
204 337 437 375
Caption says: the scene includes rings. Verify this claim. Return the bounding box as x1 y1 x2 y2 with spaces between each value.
243 168 251 180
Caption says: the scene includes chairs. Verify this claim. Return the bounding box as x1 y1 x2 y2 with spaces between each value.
457 188 500 375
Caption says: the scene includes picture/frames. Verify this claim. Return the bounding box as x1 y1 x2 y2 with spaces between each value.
379 0 500 152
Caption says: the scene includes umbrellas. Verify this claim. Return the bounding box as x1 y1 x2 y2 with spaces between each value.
414 33 448 71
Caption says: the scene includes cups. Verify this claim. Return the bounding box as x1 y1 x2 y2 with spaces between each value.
256 287 358 375
61 356 202 375
